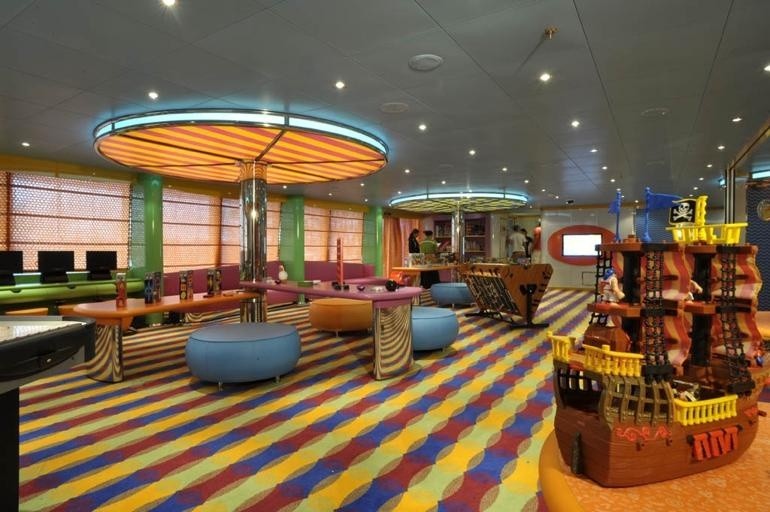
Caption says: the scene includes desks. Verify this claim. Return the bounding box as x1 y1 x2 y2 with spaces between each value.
239 280 427 381
538 399 770 512
392 264 460 306
0 278 145 304
73 289 261 383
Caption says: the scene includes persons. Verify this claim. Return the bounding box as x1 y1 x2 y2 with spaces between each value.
418 231 440 289
506 225 529 258
408 228 420 253
520 227 533 256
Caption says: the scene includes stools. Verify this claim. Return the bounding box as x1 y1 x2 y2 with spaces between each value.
185 322 301 391
430 282 476 309
411 304 460 353
309 298 373 338
59 297 137 334
6 307 48 316
